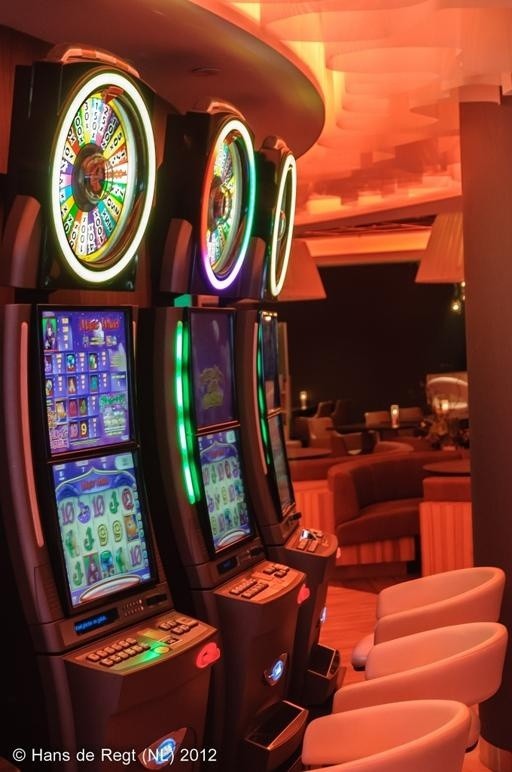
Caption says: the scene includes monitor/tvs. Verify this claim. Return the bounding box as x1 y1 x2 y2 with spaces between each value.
195 423 256 560
259 310 283 417
35 303 137 460
182 306 239 432
46 446 159 618
266 412 296 518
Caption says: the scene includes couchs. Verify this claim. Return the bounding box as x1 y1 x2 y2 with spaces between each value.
286 439 416 481
327 448 462 548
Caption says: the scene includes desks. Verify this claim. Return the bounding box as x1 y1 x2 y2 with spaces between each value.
422 460 471 477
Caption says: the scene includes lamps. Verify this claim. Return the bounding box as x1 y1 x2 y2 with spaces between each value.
276 240 327 303
413 210 464 284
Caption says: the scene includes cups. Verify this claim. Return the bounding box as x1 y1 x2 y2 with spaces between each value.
299 390 308 409
390 404 400 429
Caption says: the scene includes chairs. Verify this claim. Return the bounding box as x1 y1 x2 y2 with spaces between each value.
300 699 472 772
351 565 507 671
331 621 509 754
